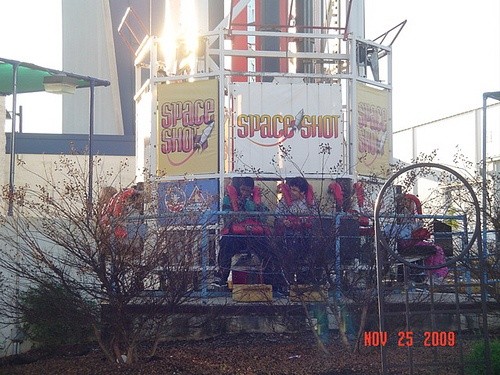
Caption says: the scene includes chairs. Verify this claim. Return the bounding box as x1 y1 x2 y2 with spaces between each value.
222 183 435 254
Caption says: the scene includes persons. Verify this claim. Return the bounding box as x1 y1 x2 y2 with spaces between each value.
397 194 453 286
274 178 332 285
212 178 287 294
